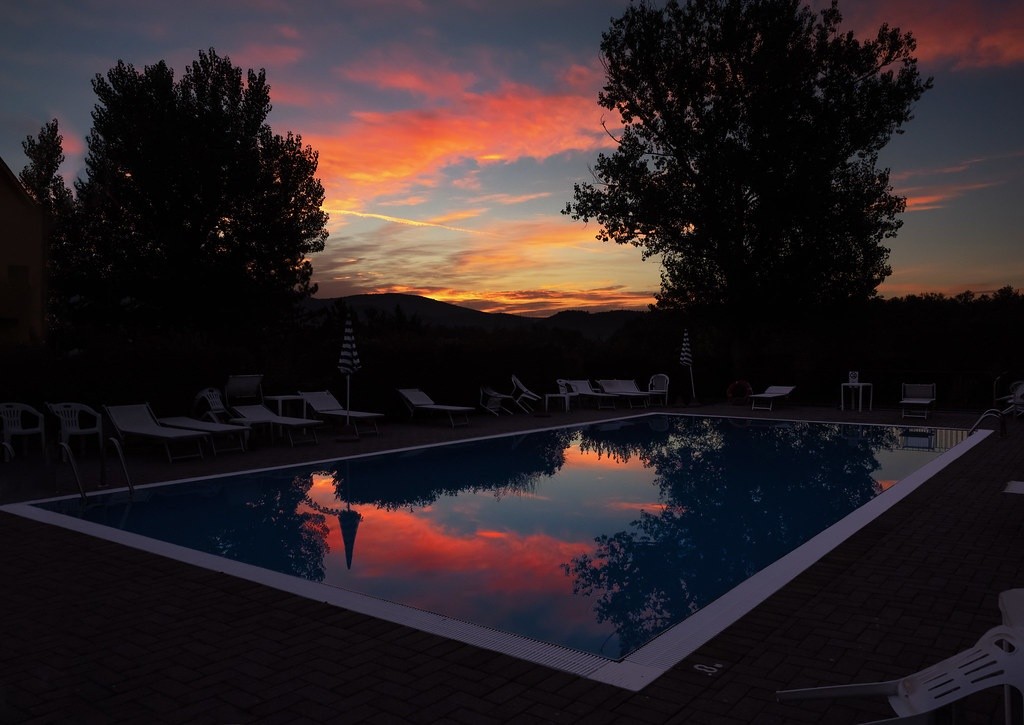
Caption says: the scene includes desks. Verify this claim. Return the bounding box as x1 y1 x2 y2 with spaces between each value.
841 382 874 411
997 589 1024 725
264 395 308 440
545 393 571 414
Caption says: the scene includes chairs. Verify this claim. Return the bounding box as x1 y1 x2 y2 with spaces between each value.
558 371 670 412
156 418 250 458
509 374 542 416
775 626 1024 725
46 402 102 462
0 400 46 466
1000 383 1024 418
479 388 513 417
900 382 937 420
298 390 385 439
748 385 797 411
103 402 209 465
195 388 231 423
232 405 324 449
399 388 475 430
